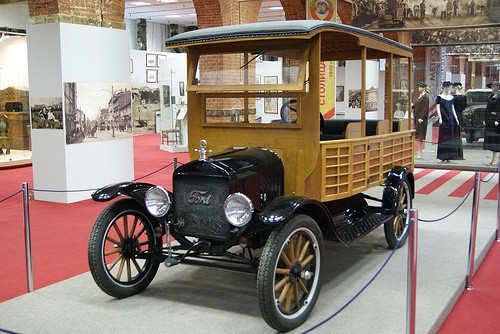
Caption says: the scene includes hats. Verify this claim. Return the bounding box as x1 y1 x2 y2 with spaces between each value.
487 81 500 89
417 82 427 86
453 82 462 86
442 81 453 86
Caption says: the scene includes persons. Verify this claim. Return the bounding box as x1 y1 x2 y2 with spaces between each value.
350 0 476 29
484 81 500 165
436 82 467 163
37 107 59 122
414 81 428 159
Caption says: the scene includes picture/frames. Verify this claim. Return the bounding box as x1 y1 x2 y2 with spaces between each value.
157 54 167 67
255 73 261 100
263 76 279 114
146 53 156 67
147 69 158 83
336 85 344 102
179 81 184 96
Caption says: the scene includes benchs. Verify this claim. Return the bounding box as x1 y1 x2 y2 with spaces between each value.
321 119 408 141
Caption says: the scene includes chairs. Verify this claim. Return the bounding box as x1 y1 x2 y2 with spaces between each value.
162 109 182 146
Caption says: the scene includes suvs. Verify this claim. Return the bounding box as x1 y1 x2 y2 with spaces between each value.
88 20 415 332
461 89 493 143
